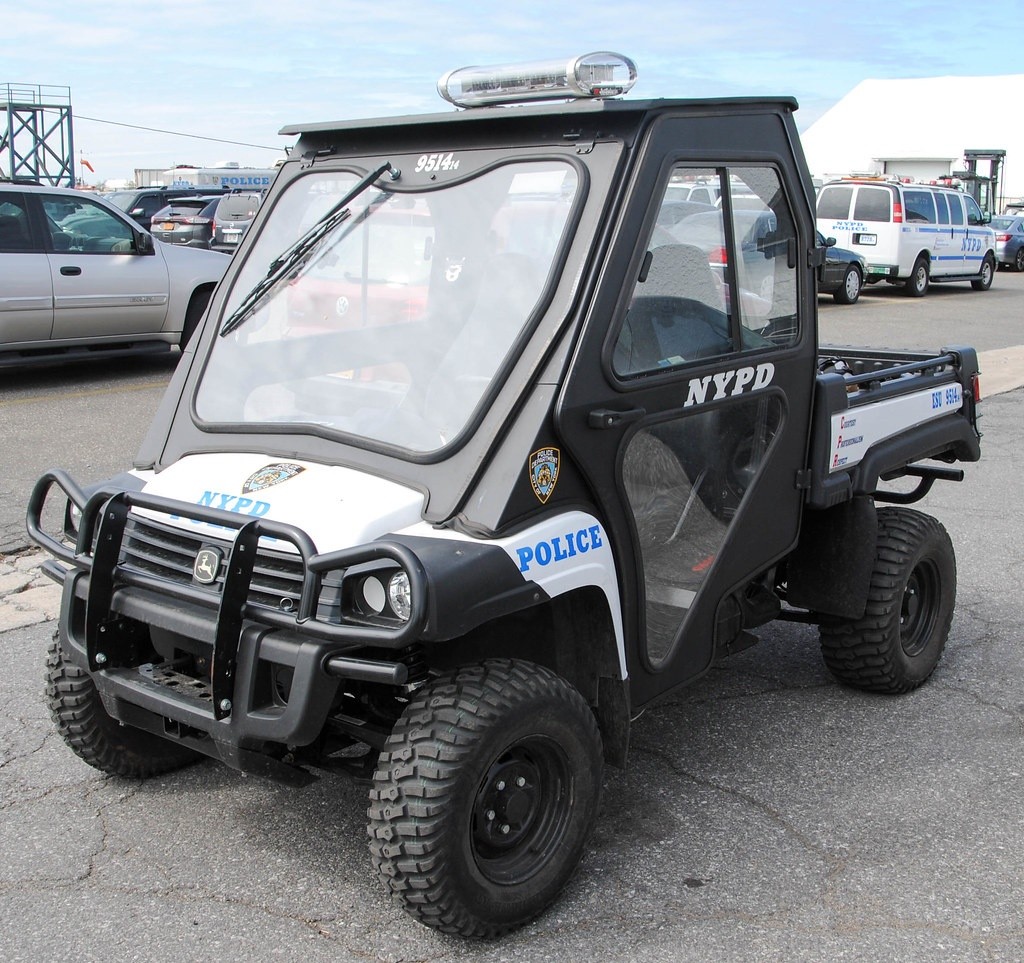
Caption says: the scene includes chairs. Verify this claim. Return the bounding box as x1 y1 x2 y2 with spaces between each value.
428 254 542 425
0 215 29 249
617 244 725 375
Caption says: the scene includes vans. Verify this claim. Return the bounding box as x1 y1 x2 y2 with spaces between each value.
814 173 1001 296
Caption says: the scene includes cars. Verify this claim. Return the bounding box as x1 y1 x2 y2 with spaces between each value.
63 163 825 418
984 215 1024 272
670 207 867 317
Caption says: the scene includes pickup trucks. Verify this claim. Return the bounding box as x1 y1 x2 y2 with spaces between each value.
20 48 985 942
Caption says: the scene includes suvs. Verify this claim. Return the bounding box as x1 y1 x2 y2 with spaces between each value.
0 182 236 367
